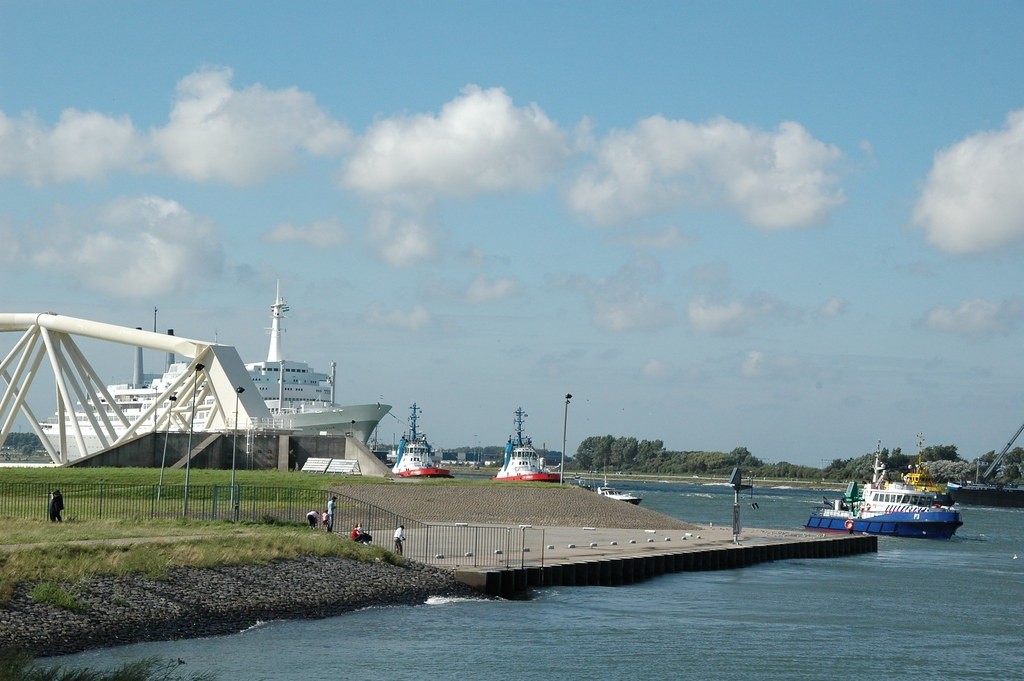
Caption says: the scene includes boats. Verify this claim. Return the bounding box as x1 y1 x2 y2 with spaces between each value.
947 422 1024 509
391 402 451 478
803 431 964 541
490 406 561 482
564 457 644 506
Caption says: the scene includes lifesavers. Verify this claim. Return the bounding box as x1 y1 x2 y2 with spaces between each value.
844 520 854 530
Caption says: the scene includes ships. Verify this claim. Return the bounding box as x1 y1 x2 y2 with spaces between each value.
35 278 393 464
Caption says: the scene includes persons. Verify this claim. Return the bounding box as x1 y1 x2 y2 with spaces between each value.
327 496 337 532
322 510 328 525
307 511 319 529
352 522 369 545
49 489 64 521
393 525 404 556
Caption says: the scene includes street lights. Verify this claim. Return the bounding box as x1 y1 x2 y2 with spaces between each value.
560 393 573 483
